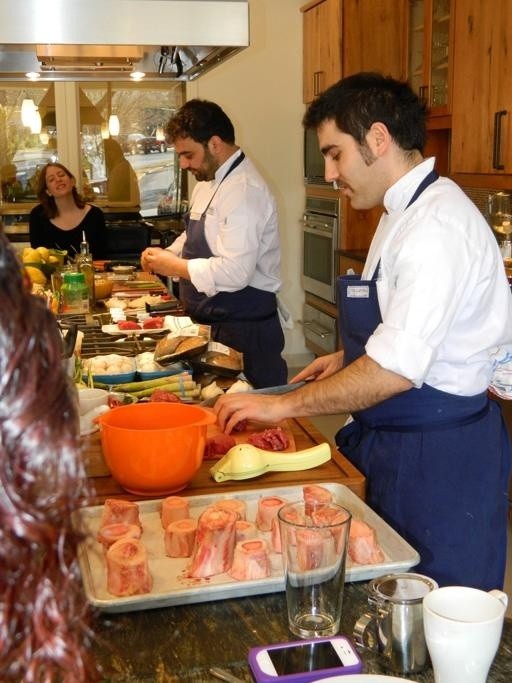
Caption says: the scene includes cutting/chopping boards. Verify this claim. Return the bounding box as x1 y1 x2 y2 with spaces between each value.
85 400 368 498
99 271 179 310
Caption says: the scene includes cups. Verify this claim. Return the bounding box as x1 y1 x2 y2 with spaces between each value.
277 500 352 641
489 194 510 238
353 571 439 674
422 585 509 683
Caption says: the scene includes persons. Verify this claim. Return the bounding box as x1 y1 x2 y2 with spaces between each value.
93 137 139 208
211 70 512 593
141 98 294 366
29 162 107 263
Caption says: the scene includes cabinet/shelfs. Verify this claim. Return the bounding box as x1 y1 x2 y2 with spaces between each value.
449 0 512 175
403 0 454 130
299 0 402 104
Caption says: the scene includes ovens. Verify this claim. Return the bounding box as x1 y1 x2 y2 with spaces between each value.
298 196 342 358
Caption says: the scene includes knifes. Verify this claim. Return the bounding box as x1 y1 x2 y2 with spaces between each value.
235 379 317 395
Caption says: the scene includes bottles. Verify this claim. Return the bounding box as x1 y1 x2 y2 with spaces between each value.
60 273 89 314
77 242 96 311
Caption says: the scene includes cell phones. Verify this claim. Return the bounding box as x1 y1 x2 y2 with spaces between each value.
248 637 361 683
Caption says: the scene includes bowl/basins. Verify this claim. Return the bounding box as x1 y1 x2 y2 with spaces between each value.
92 401 217 495
111 266 136 274
94 279 114 299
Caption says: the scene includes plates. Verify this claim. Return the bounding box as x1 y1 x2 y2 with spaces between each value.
192 352 245 381
79 357 137 386
101 323 169 335
153 325 211 364
135 355 193 381
70 481 421 613
